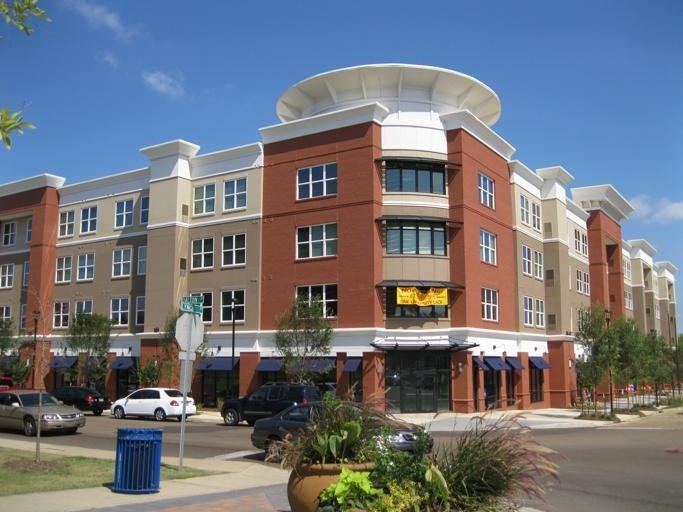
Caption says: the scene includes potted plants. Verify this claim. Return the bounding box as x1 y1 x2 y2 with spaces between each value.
265 380 564 512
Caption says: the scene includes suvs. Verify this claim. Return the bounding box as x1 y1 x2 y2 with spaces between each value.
221 382 320 428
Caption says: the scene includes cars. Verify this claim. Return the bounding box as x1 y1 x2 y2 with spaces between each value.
0 389 85 437
247 401 436 467
107 387 198 423
51 385 113 419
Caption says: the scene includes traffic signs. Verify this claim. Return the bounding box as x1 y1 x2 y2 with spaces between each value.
180 302 203 315
180 294 206 304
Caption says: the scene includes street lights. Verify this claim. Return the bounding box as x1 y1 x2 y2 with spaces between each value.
30 310 40 389
603 308 616 418
227 296 237 401
649 327 661 408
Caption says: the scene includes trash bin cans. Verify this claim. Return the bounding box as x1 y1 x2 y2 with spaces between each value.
112 428 164 495
